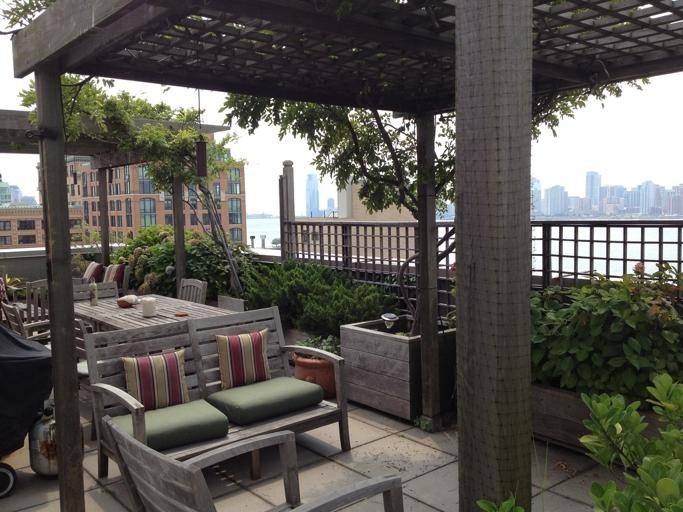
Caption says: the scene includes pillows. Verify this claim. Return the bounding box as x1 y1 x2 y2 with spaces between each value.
120 346 192 414
214 326 275 389
82 260 125 285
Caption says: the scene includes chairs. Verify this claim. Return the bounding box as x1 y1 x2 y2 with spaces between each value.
2 277 251 362
98 414 407 512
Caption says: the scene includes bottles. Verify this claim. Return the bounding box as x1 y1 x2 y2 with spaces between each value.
88 276 98 307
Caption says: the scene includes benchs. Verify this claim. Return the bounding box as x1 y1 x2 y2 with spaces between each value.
79 303 355 482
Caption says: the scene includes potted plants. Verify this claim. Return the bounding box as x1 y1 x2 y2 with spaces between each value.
290 334 345 398
